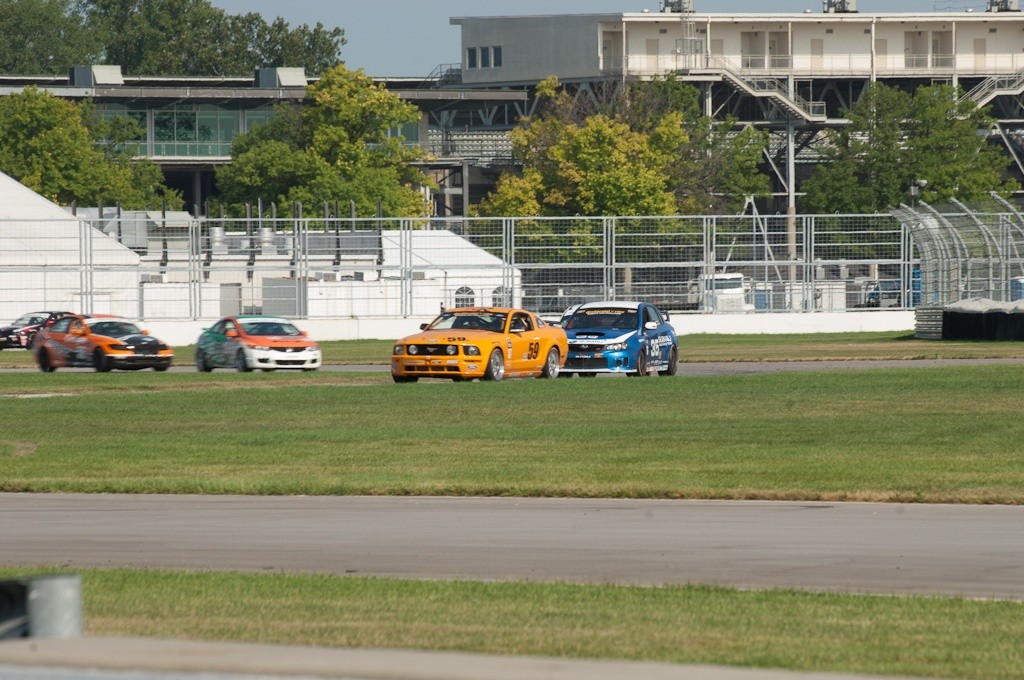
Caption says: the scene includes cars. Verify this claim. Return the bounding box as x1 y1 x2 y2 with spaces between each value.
555 300 679 378
0 310 75 350
33 315 174 373
390 306 569 383
197 315 323 371
